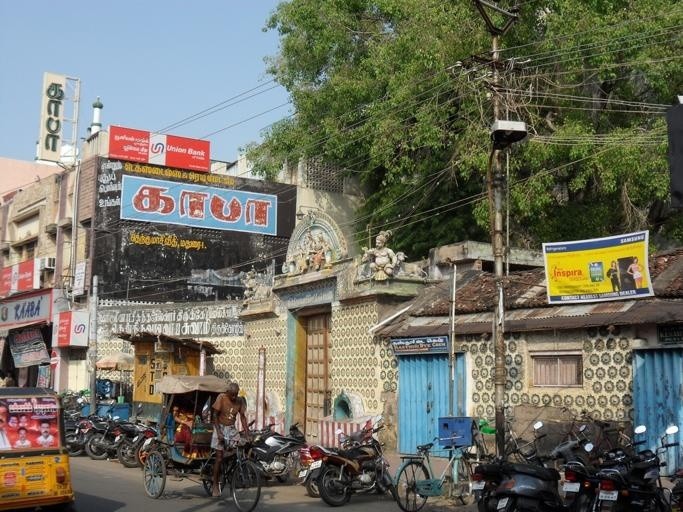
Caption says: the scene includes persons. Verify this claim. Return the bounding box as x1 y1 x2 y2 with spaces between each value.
4 370 15 387
19 415 27 427
173 392 210 459
607 261 621 292
14 427 32 449
363 230 397 280
0 419 11 450
627 256 644 288
211 383 251 497
242 271 256 307
9 416 18 427
37 419 54 447
0 369 6 387
312 232 334 271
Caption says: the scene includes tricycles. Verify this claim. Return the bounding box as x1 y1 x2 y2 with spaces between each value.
143 376 262 511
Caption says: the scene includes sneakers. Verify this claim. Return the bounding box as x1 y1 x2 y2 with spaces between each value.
212 485 221 496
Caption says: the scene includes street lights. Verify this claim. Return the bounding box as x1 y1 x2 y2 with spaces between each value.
490 120 530 461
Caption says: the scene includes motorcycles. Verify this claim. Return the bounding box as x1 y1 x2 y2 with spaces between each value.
65 398 157 469
225 423 309 489
559 425 645 511
473 421 551 512
298 416 373 498
494 424 590 512
308 415 399 506
593 426 680 511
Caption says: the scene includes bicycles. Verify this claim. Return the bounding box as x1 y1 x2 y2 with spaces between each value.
393 433 473 512
439 405 535 478
560 408 634 462
